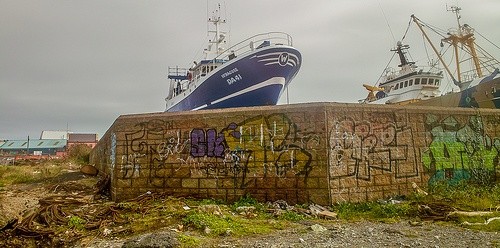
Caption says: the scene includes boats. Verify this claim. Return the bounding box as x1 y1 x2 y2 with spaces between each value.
164 0 304 113
357 21 444 104
